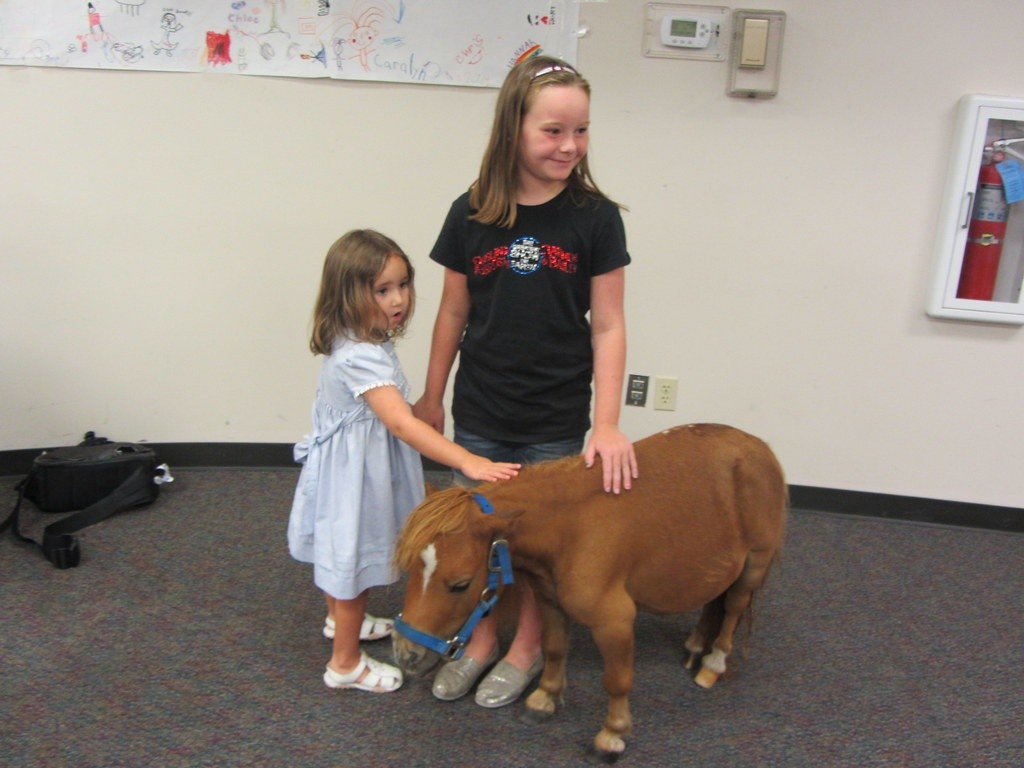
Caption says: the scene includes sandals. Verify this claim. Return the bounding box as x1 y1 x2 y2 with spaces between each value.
324 653 403 694
321 613 392 641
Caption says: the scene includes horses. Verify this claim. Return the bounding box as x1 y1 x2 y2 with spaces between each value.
391 422 788 766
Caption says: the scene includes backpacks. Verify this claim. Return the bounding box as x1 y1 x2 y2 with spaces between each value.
0 430 161 568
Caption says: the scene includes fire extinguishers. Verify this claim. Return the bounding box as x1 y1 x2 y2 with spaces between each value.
958 138 1024 301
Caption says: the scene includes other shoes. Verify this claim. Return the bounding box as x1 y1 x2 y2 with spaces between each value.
475 654 544 708
434 639 499 701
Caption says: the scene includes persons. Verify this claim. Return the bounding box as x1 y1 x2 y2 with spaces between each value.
285 229 522 692
409 57 639 707
